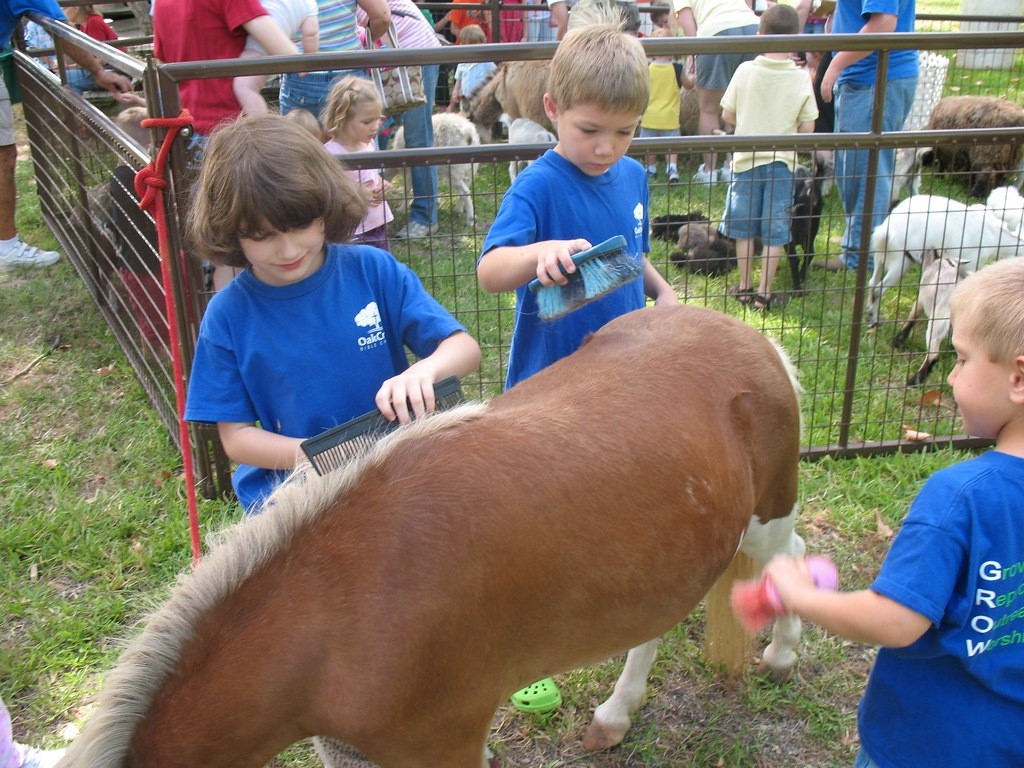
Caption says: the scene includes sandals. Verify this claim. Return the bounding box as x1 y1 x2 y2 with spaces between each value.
727 282 753 306
750 290 790 313
511 677 563 715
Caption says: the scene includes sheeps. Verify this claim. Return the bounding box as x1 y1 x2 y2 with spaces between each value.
866 185 1024 386
649 159 827 299
916 95 1024 199
672 66 724 171
380 111 482 224
454 57 575 184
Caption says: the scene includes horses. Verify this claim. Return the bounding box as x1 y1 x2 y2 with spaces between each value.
48 302 807 768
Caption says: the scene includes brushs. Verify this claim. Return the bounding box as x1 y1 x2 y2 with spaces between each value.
730 554 839 632
527 236 642 323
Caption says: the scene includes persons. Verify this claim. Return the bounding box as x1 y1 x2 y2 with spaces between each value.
0 0 920 309
188 114 483 768
475 27 683 716
746 256 1024 768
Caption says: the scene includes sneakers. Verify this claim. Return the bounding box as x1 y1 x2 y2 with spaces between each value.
718 167 734 184
691 162 718 186
0 241 61 268
394 221 439 242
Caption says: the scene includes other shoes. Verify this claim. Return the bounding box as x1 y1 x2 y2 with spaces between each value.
645 166 658 178
664 167 680 182
76 123 91 140
812 256 850 274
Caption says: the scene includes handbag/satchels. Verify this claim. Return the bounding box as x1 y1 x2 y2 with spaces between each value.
357 20 429 119
